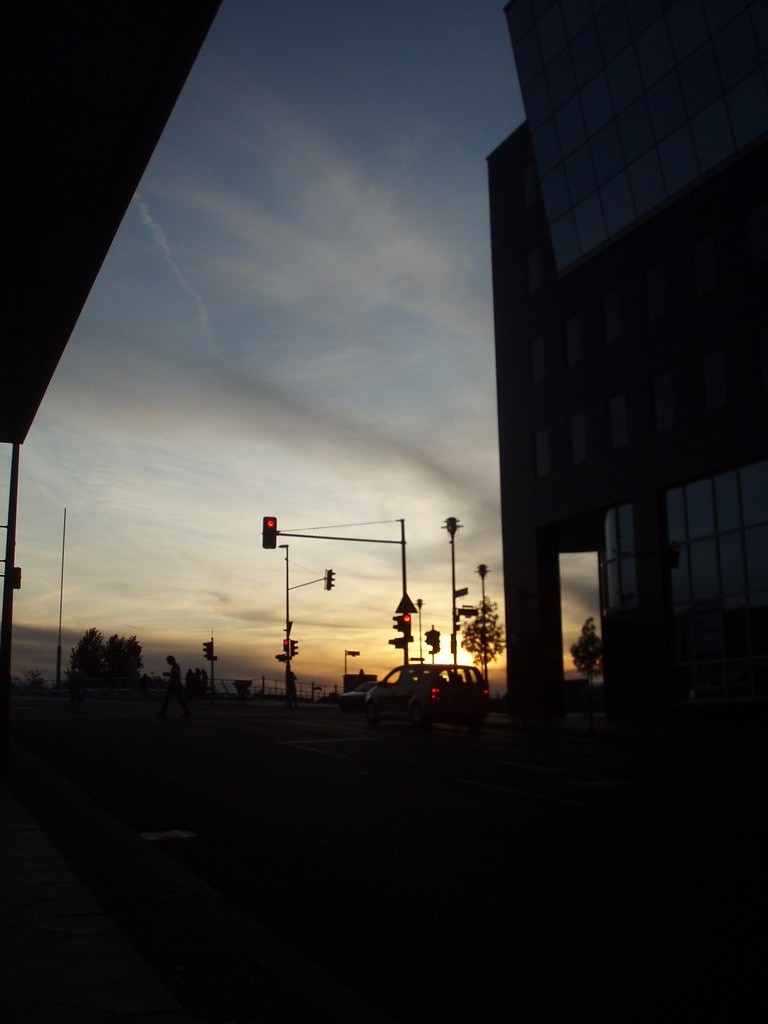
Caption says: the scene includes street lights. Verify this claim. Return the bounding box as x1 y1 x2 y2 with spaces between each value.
441 517 462 675
416 599 423 663
475 564 488 682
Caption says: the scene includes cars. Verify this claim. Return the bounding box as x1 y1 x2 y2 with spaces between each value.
336 681 380 711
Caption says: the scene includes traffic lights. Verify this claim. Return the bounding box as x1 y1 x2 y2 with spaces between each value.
324 569 336 590
284 639 288 652
262 516 277 548
275 655 287 663
388 637 406 648
392 616 411 633
288 639 298 658
203 642 212 661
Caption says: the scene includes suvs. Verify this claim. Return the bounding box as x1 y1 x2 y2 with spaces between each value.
364 663 491 733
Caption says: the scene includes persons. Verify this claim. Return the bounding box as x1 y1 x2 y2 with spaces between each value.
156 656 190 719
